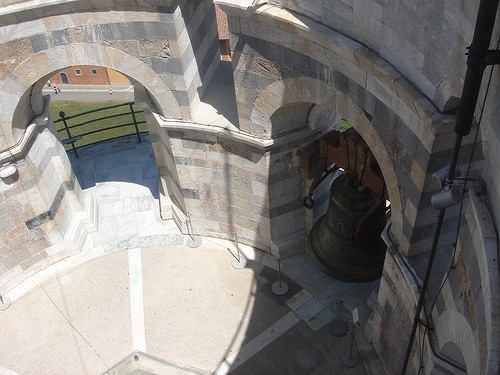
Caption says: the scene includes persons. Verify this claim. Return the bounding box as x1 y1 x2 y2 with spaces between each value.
48 79 61 96
109 86 113 95
